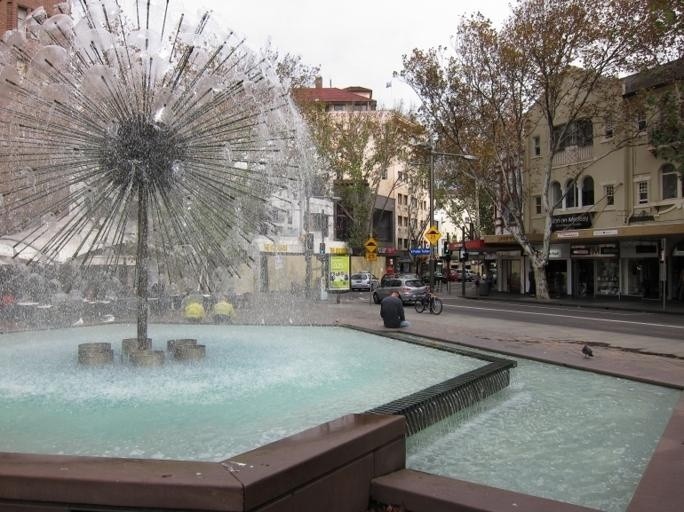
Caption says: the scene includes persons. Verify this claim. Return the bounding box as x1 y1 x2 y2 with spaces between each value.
184 298 206 322
212 295 235 324
380 291 405 328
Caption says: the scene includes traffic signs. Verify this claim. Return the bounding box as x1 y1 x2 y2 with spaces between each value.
409 249 431 255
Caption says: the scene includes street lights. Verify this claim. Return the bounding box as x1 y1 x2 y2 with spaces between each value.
305 194 345 299
407 148 479 294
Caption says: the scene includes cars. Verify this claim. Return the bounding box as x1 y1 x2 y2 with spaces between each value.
350 263 497 305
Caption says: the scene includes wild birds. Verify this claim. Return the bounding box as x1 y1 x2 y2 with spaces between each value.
582 344 595 359
72 317 84 328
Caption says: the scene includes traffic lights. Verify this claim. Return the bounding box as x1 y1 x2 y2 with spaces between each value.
458 247 469 262
443 242 452 256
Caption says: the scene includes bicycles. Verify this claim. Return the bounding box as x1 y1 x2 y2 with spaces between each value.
413 287 443 316
